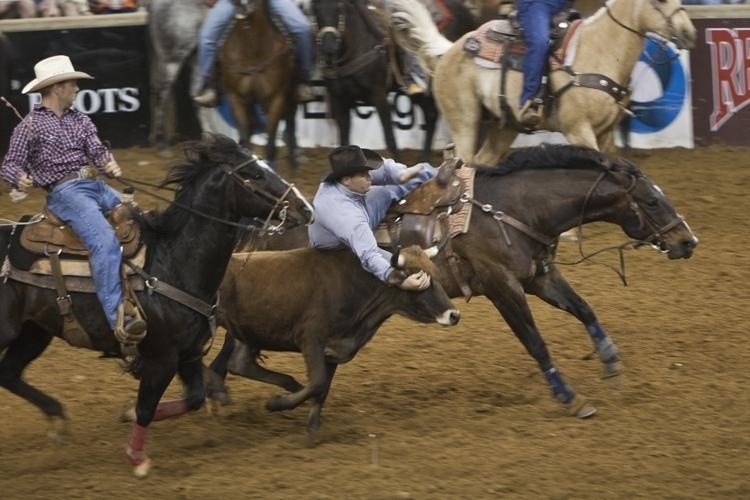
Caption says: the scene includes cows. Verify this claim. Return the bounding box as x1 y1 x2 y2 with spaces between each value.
206 232 461 429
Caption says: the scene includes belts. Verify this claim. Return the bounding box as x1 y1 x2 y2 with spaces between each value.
42 165 96 193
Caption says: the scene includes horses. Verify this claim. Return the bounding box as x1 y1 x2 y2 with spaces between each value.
0 131 317 478
207 144 701 419
311 1 441 166
216 1 302 162
387 0 699 167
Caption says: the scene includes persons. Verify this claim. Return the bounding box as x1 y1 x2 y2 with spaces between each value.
1 55 146 353
386 0 428 94
306 146 438 292
0 0 140 20
516 0 570 123
191 0 316 107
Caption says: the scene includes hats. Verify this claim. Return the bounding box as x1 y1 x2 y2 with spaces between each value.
21 55 96 95
321 146 384 185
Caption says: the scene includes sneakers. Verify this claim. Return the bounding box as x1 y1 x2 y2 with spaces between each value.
403 79 424 96
297 83 315 102
192 92 218 107
521 107 541 125
123 317 146 335
